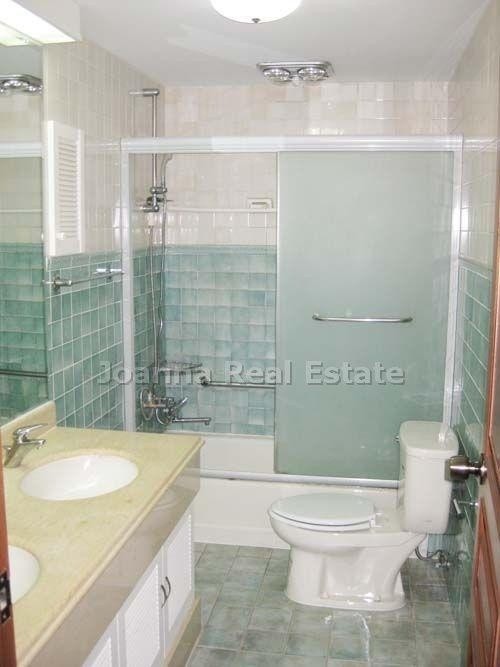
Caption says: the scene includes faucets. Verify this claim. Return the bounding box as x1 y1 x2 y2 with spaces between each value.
173 418 212 426
4 425 49 468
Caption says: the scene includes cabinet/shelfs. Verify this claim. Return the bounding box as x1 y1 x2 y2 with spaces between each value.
121 512 194 667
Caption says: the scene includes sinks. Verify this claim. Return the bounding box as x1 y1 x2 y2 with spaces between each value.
19 454 139 502
8 545 39 603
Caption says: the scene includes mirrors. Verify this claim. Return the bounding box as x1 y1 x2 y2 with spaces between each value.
0 21 52 428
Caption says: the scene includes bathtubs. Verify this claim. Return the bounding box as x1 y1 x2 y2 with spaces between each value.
165 429 429 559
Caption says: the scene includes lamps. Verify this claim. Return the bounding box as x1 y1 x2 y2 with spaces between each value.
210 0 303 24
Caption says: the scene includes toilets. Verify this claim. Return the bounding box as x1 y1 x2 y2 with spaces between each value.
267 421 459 612
